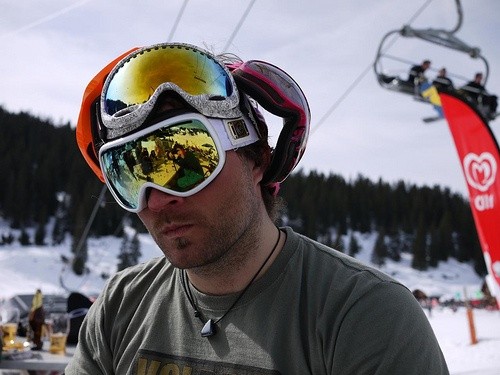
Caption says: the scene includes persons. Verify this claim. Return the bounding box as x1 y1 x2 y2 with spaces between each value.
64 41 450 375
406 59 489 93
98 142 205 193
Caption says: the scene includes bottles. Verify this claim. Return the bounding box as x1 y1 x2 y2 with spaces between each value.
29 289 45 350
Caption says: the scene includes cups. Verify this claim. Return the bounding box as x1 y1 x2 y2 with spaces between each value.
1 308 18 347
48 314 70 355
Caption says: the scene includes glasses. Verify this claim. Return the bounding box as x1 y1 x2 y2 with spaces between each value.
224 59 311 187
101 42 240 139
98 112 261 213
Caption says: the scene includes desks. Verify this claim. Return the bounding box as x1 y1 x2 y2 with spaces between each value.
0 336 77 375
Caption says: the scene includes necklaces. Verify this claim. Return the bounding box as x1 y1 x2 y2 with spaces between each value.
177 224 282 338
